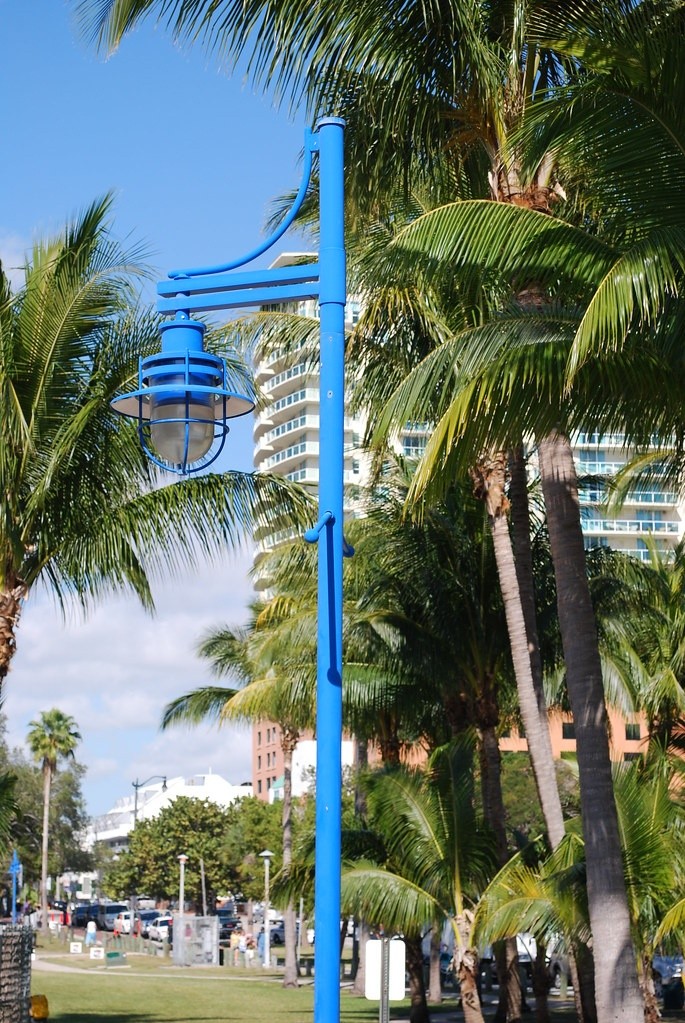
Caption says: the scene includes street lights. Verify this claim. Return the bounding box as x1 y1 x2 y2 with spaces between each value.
259 850 276 968
131 774 167 831
176 854 189 914
7 849 21 927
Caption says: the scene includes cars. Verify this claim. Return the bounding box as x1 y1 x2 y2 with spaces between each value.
105 109 345 1022
339 913 405 942
647 945 685 1010
19 892 266 967
256 918 314 947
414 904 574 997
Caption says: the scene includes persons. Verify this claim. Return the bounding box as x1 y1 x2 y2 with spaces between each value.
70 900 75 913
86 921 97 947
229 927 265 967
0 882 42 928
